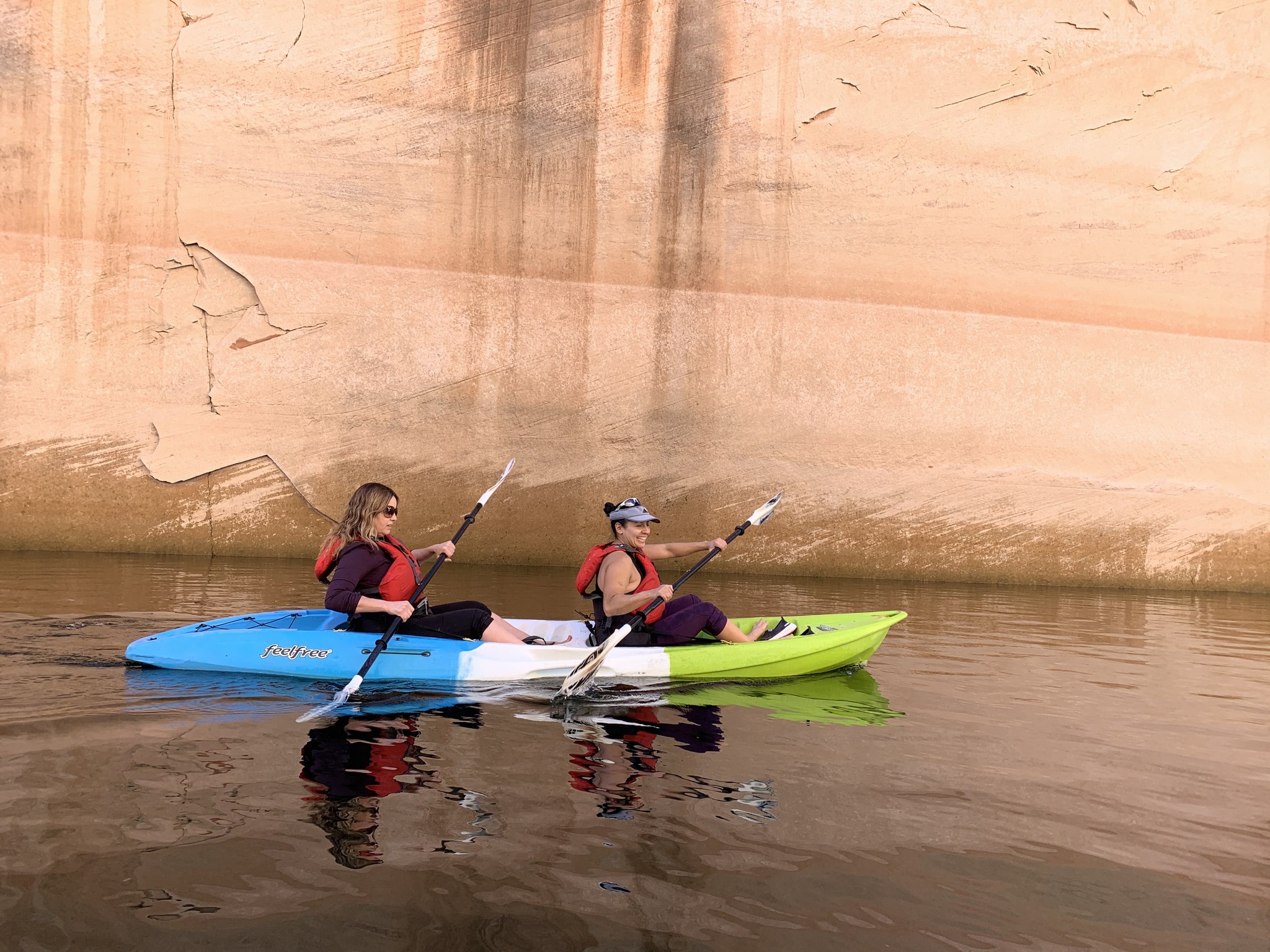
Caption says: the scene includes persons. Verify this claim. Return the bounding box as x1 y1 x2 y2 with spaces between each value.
575 498 770 647
315 483 572 646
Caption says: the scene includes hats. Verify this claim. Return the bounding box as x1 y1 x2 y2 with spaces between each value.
609 506 660 523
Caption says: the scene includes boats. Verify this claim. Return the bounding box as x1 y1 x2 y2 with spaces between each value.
125 609 907 684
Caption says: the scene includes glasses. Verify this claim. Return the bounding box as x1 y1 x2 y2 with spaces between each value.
615 498 640 511
383 506 398 518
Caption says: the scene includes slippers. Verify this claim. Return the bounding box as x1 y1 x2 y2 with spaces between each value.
522 636 555 645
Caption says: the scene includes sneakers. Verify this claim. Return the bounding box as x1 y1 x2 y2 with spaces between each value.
799 626 816 635
760 616 797 641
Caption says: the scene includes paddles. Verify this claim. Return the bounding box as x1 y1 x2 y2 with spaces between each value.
559 488 788 696
339 456 518 698
